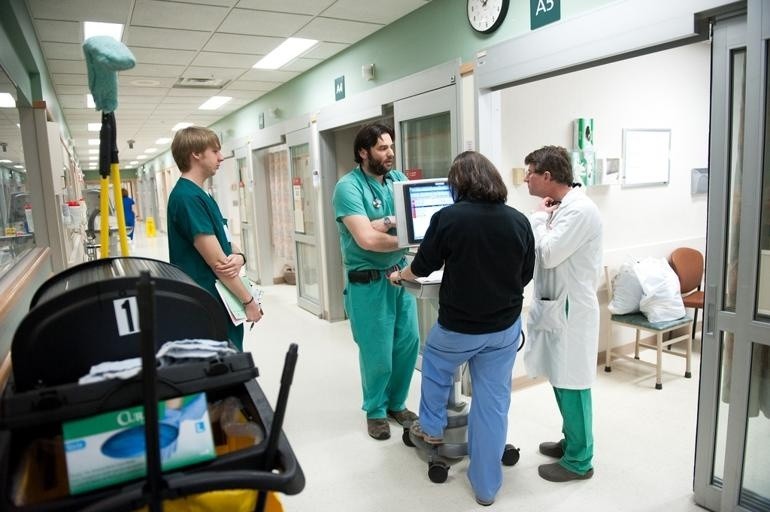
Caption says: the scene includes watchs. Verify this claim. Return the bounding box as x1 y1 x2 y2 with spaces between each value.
384 217 391 230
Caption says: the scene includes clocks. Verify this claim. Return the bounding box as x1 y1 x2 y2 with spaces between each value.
463 0 509 35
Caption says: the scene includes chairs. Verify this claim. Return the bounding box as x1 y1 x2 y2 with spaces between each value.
665 247 702 348
601 263 696 391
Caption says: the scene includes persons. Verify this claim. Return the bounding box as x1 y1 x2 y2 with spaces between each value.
390 152 535 506
332 124 419 440
166 128 262 357
525 145 605 482
121 188 135 240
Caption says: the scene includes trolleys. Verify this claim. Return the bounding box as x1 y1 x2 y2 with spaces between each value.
398 267 520 483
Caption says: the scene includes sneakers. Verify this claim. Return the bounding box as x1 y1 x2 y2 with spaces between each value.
367 418 390 440
387 408 419 425
538 462 593 482
410 420 445 445
540 442 564 458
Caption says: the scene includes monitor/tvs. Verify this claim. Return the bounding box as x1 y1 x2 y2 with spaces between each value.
392 177 454 248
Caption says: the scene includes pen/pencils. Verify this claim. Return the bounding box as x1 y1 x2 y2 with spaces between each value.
250 303 261 332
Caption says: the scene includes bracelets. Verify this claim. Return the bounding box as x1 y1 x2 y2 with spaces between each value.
399 270 402 279
243 297 253 305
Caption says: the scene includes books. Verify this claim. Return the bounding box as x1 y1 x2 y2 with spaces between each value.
214 277 260 326
